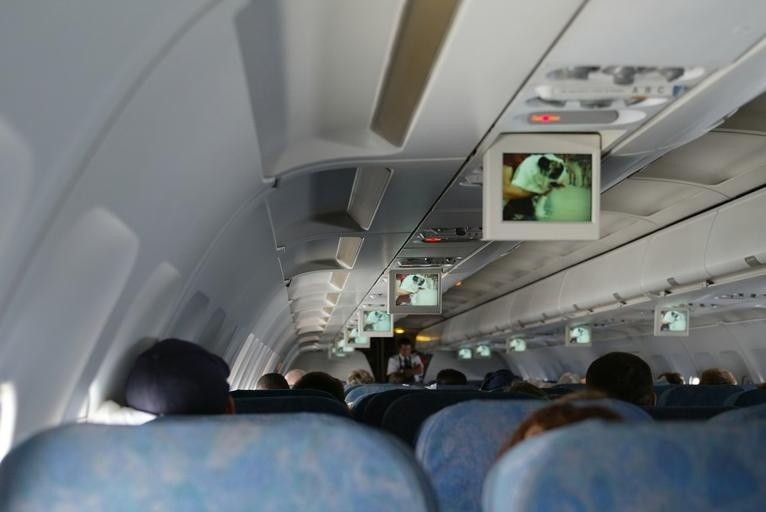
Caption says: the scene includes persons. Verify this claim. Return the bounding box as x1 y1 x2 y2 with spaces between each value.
284 368 308 390
292 372 345 397
657 372 686 384
503 153 569 221
256 373 290 390
387 340 425 385
735 386 765 407
586 351 662 419
700 368 738 386
557 372 582 385
505 389 625 463
436 368 467 385
396 274 429 305
481 368 537 391
346 369 373 385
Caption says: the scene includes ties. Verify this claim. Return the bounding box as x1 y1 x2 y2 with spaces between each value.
405 358 408 367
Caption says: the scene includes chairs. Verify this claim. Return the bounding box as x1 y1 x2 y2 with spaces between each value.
0 376 765 509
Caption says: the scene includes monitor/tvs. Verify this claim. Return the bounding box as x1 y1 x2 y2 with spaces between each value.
474 341 492 359
482 132 601 242
329 325 370 359
358 307 394 337
388 267 443 315
505 334 528 354
654 306 689 336
456 345 473 360
565 322 592 347
